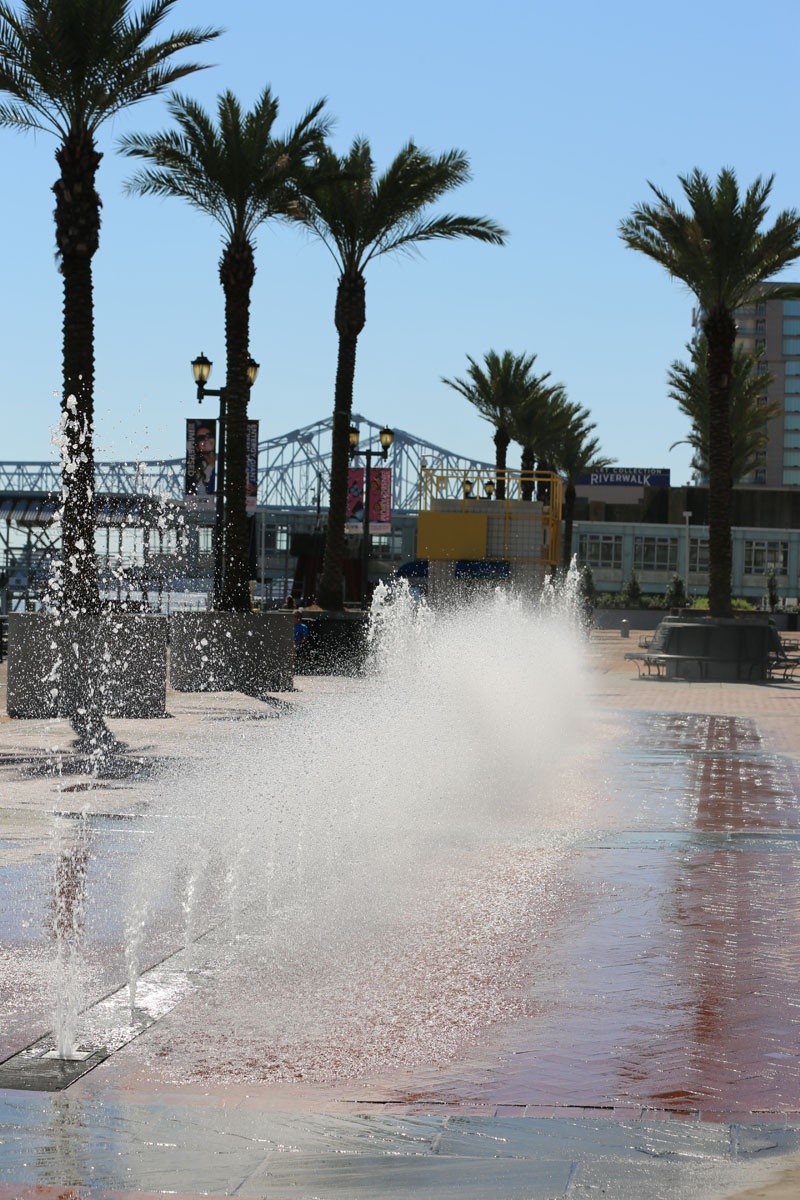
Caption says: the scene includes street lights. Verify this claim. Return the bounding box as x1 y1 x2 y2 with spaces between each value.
190 349 260 604
346 421 395 605
683 511 693 599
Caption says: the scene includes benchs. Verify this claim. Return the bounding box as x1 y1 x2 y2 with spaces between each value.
623 633 800 684
